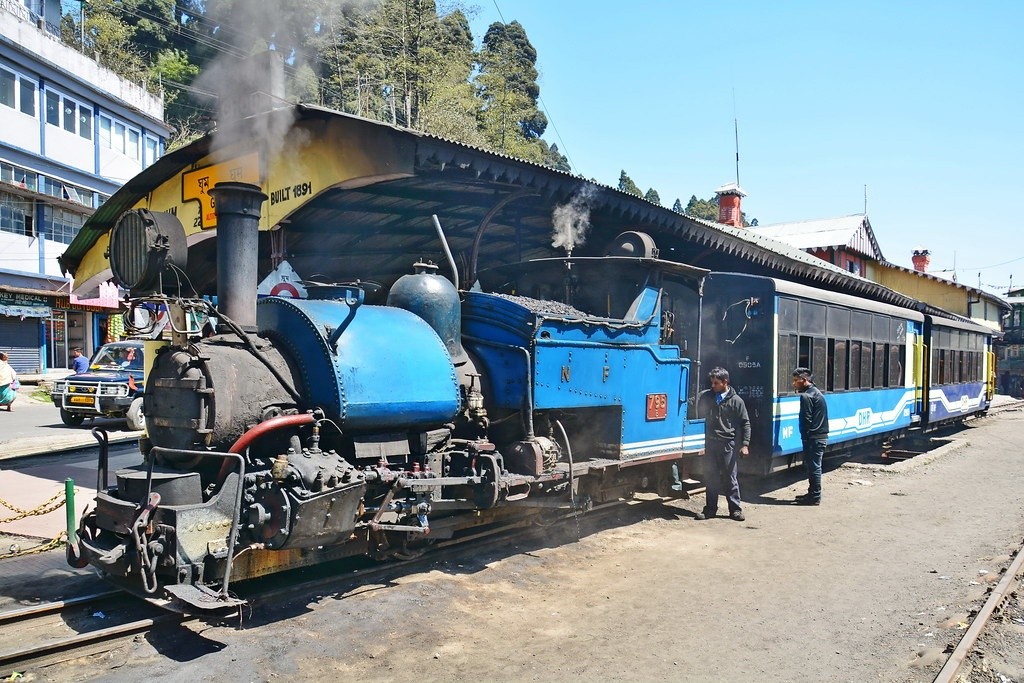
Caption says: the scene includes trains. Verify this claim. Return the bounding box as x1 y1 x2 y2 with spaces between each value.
58 177 1004 627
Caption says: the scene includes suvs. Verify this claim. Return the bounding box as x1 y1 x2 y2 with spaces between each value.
49 338 158 431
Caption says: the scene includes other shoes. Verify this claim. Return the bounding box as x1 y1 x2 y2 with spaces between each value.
794 494 820 506
728 509 745 520
695 507 716 519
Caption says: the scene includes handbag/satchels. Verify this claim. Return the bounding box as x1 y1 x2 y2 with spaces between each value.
11 378 19 389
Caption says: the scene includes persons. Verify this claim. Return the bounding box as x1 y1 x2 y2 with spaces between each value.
0 352 16 411
73 347 89 374
687 368 751 521
793 367 829 505
